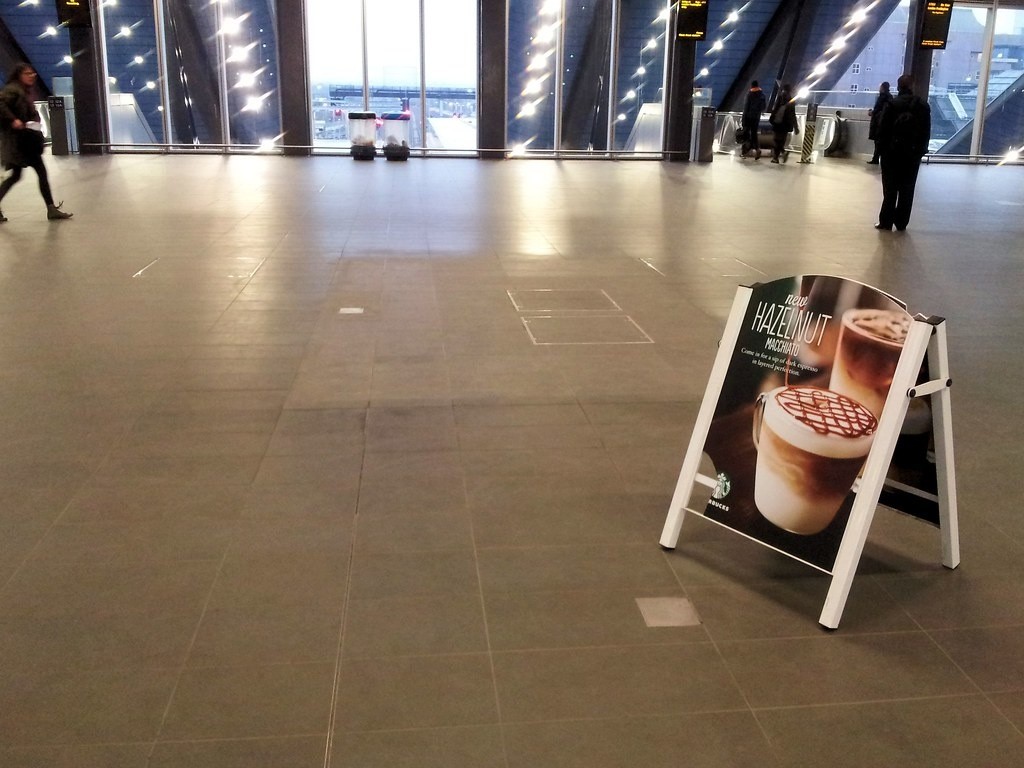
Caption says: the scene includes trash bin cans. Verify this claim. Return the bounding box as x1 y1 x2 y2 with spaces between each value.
348 113 377 161
381 113 410 161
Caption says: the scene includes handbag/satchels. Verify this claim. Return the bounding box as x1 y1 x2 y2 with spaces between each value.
735 128 749 143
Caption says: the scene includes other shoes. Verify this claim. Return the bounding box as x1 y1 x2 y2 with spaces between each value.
771 158 779 163
867 158 879 164
740 154 746 159
875 223 892 230
755 148 761 160
47 201 73 220
0 210 7 221
781 151 788 163
897 227 906 231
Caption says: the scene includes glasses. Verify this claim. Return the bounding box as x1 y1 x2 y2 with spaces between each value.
24 71 37 77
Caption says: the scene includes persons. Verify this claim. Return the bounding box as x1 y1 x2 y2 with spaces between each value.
874 75 931 230
866 81 894 164
0 62 73 222
739 80 767 161
769 84 799 164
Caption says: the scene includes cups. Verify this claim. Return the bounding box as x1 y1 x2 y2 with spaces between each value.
829 309 903 420
752 385 878 535
